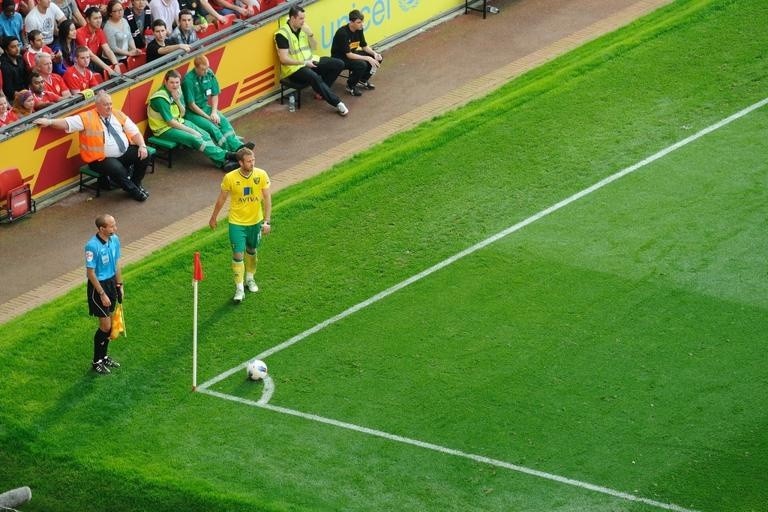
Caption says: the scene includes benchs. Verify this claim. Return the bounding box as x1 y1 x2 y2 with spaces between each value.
280 68 352 109
80 145 157 197
147 136 180 168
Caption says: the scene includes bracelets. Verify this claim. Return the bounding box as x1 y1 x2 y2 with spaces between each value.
98 289 104 294
263 220 270 225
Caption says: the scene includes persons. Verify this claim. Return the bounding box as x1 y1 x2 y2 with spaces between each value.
332 9 383 96
1 1 259 124
146 69 241 172
181 55 256 151
84 212 124 374
274 5 349 116
208 146 272 301
32 92 150 201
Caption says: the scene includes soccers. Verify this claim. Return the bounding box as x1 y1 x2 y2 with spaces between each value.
247 359 267 381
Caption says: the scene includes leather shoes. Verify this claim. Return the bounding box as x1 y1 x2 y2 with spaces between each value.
132 186 149 202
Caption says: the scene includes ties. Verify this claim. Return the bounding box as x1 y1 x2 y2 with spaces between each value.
105 118 126 153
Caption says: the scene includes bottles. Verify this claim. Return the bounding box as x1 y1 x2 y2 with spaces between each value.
288 93 296 113
369 62 379 75
486 5 499 14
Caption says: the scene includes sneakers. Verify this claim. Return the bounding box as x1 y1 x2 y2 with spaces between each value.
234 288 245 301
337 102 348 116
92 360 110 374
346 80 375 95
247 279 258 292
224 141 255 172
104 356 120 367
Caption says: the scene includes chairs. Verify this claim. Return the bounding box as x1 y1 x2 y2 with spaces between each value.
7 183 36 223
0 0 285 90
